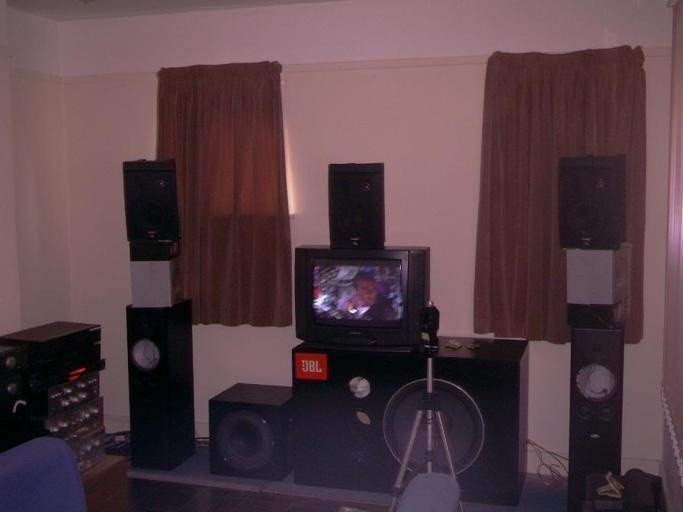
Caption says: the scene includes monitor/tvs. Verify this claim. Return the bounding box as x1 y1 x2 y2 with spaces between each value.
294 245 430 353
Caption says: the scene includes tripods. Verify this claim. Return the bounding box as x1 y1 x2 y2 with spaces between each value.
387 354 465 512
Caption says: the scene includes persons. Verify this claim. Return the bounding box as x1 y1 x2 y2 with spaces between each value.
353 271 395 320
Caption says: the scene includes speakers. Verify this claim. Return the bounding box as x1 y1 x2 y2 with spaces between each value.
123 160 178 242
567 320 625 512
329 163 385 249
209 383 293 482
126 297 195 471
559 153 626 250
